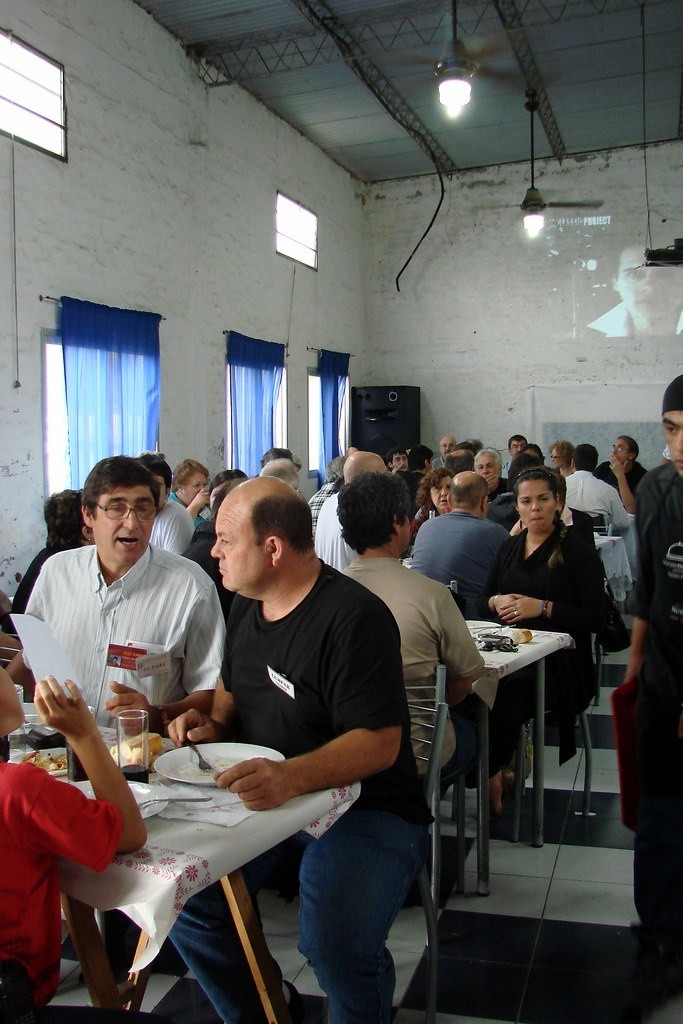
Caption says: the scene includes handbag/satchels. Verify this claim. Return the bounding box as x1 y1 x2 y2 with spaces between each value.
596 574 630 652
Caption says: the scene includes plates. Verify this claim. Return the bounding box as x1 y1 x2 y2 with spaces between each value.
154 742 286 787
465 620 502 638
9 748 67 776
69 779 169 819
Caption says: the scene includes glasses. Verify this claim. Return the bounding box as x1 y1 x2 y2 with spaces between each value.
96 502 159 520
550 455 563 459
612 446 628 452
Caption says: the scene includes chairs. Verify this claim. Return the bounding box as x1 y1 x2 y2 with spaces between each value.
406 520 616 1022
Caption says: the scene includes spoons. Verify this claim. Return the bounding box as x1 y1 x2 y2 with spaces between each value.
186 739 212 771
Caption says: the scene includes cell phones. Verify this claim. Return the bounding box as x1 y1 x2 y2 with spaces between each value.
26 723 66 749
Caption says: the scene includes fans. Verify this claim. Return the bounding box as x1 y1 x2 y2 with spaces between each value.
383 0 538 84
471 87 603 210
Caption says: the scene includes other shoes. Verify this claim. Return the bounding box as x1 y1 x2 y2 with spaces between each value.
284 980 306 1024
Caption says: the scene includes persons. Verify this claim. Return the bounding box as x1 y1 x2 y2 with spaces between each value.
408 470 513 621
0 664 148 1024
462 467 605 818
335 472 485 780
11 489 96 615
5 455 226 738
623 374 683 996
108 656 118 665
137 435 647 572
168 477 436 1024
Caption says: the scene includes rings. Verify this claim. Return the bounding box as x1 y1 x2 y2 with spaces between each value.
514 611 518 616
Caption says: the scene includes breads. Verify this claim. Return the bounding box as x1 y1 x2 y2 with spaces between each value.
109 732 163 767
513 629 532 643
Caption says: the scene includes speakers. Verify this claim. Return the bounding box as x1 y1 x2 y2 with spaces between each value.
352 386 420 465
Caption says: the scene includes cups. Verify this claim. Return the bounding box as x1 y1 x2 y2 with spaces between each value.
115 709 150 784
8 684 25 736
65 705 95 783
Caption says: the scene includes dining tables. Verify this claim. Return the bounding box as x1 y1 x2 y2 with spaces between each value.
455 616 575 897
8 721 362 1024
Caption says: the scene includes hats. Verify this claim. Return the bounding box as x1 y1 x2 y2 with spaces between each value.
662 375 683 414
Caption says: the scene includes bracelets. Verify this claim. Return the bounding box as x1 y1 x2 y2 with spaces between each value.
541 600 549 615
154 704 170 738
494 595 499 599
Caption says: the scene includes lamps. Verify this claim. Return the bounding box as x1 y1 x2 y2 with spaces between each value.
523 207 546 240
437 76 472 114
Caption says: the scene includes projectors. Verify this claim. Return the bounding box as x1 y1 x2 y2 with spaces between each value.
644 250 683 267
